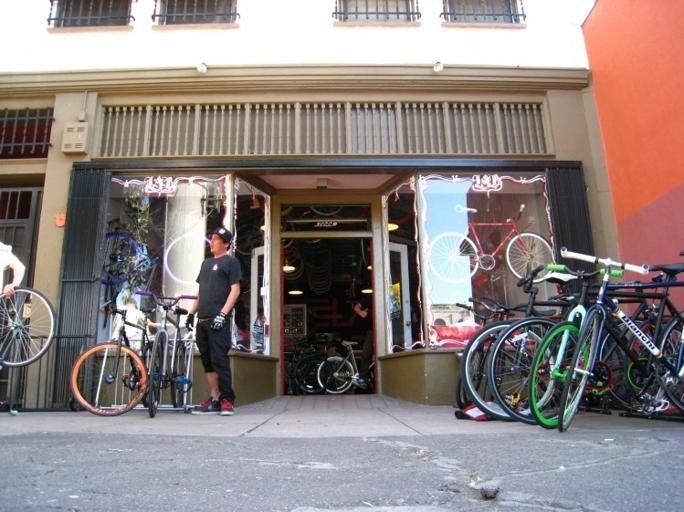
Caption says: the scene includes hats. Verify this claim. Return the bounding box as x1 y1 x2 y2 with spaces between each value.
206 226 232 247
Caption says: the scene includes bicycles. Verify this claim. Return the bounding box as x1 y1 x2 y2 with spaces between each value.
69 290 162 417
427 204 555 285
100 206 213 286
0 287 57 371
135 290 198 418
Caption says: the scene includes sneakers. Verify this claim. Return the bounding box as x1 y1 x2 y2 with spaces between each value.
221 398 234 416
188 396 220 415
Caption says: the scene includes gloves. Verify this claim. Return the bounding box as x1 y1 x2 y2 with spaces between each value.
186 314 195 331
211 313 225 329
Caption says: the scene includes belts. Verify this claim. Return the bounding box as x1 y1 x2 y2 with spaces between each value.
197 318 214 323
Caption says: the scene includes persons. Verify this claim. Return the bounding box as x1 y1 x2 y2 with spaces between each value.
354 279 373 389
430 319 450 346
0 239 26 299
184 228 244 416
110 297 162 356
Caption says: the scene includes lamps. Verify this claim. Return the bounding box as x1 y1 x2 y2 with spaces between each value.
282 258 297 274
194 62 209 73
431 60 444 75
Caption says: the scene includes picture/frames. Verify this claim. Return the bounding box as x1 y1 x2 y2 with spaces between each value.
283 303 308 337
